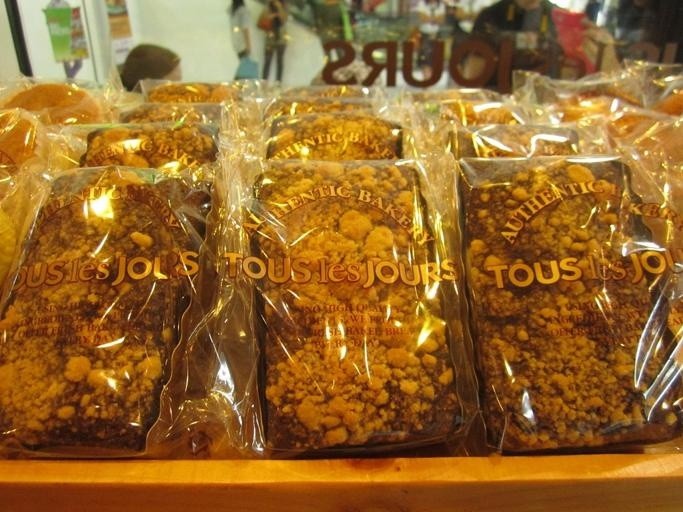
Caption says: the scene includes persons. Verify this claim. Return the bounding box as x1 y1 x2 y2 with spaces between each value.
465 0 578 94
448 0 489 90
313 0 353 64
227 0 259 79
411 0 445 88
256 0 287 81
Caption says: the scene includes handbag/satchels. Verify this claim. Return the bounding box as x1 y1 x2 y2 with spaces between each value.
257 10 273 32
235 56 258 80
